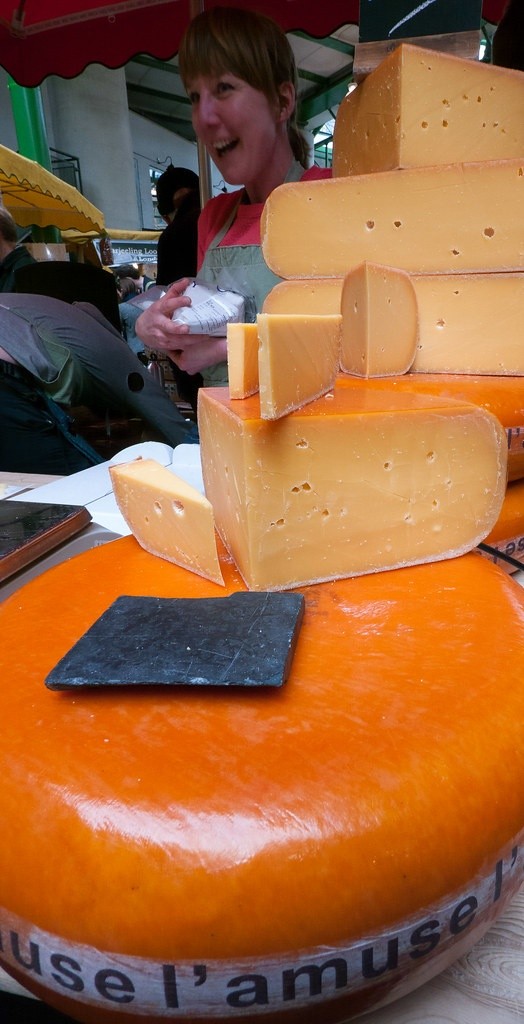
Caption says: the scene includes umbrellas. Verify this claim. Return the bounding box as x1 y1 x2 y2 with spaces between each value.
0 144 105 234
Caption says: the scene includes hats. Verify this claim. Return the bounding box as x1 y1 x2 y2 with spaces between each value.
156 165 201 215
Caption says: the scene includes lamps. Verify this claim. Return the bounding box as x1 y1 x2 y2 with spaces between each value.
213 180 227 193
157 157 175 171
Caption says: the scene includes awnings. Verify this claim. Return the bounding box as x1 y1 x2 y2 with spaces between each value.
59 228 163 271
1 0 513 90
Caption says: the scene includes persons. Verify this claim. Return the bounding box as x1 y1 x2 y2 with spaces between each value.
0 207 40 378
155 163 201 417
136 6 333 390
117 265 204 413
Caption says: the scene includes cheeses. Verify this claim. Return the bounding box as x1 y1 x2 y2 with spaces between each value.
103 46 524 596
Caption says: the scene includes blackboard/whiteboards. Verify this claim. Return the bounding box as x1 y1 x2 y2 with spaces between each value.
93 238 158 266
